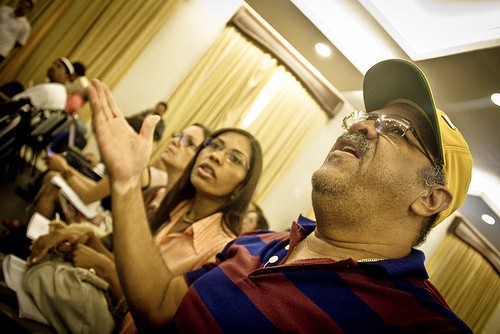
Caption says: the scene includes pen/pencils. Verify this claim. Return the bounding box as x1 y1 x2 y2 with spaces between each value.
56 212 60 222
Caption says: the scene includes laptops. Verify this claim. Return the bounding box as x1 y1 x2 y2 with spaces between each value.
68 119 92 164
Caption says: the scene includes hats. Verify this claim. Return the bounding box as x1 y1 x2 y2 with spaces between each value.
363 58 474 227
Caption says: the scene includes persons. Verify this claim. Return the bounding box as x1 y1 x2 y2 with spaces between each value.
87 58 474 334
0 57 269 334
0 0 34 61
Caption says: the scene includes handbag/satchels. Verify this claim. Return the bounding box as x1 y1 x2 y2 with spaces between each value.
21 256 114 334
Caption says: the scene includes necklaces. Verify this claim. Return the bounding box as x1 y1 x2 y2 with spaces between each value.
356 257 390 263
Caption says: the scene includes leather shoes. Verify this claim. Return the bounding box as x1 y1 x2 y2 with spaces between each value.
1 215 22 229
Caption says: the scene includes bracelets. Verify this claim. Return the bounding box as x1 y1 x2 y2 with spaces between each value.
61 168 75 179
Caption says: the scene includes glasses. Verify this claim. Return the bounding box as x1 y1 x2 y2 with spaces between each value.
342 108 437 170
202 135 251 169
171 130 199 148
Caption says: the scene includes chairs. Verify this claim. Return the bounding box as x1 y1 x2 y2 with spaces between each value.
0 105 75 182
